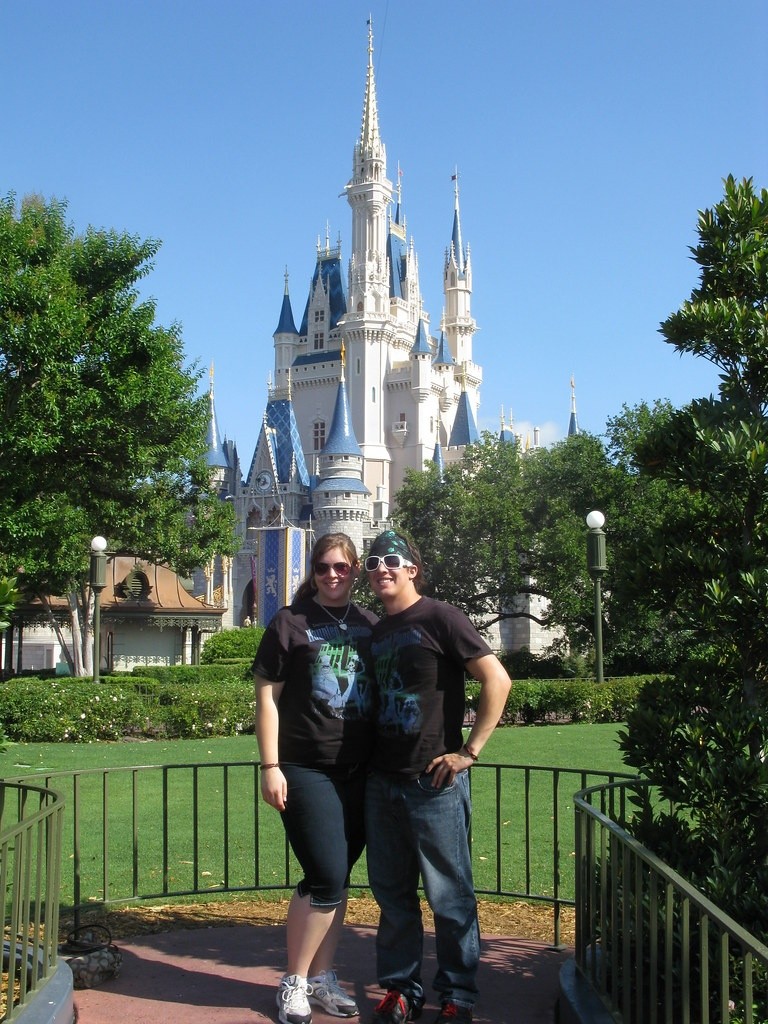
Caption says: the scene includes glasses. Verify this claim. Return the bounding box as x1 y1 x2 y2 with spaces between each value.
365 554 413 572
314 561 353 576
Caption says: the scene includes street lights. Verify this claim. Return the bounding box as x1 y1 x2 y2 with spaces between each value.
88 536 108 684
586 511 609 681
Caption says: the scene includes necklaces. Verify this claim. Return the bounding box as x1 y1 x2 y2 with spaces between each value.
320 597 350 631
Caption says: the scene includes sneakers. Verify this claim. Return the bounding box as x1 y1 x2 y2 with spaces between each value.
308 971 360 1017
373 989 422 1024
436 1002 473 1024
277 972 313 1024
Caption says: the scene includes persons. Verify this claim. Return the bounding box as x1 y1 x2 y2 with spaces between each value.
364 528 512 1024
249 533 380 1023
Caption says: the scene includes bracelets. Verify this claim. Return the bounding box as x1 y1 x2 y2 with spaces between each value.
463 744 479 761
260 763 280 770
